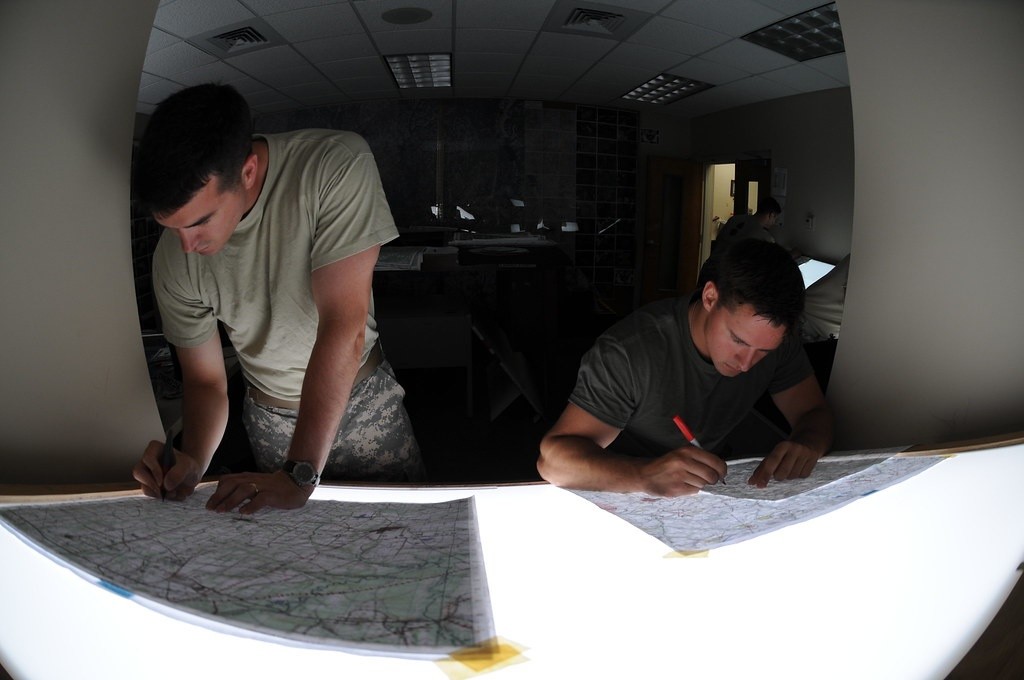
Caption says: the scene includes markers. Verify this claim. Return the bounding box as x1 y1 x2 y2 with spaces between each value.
672 414 727 485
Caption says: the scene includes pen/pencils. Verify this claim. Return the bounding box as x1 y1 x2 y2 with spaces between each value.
157 430 174 502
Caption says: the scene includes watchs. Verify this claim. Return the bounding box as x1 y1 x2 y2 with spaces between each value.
281 460 321 489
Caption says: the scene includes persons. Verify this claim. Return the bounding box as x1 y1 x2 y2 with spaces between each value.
536 239 831 498
710 198 781 255
132 83 429 515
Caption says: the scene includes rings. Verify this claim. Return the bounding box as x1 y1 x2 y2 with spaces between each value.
249 482 258 493
